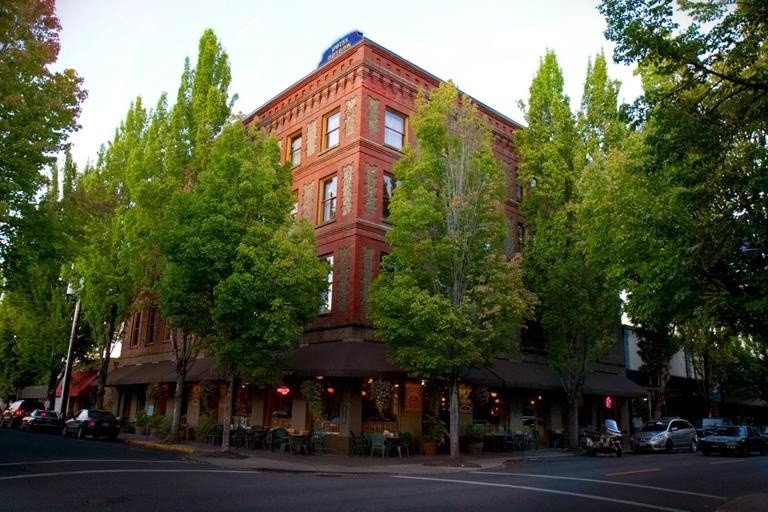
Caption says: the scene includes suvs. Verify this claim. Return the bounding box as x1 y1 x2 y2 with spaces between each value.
0 399 45 431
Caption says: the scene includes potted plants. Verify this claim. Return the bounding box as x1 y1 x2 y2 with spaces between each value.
459 421 485 456
418 413 446 457
133 409 165 437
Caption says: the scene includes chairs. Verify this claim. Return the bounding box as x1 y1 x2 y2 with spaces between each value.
350 426 415 459
207 422 328 453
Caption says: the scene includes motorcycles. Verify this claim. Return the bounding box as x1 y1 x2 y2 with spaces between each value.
580 418 628 458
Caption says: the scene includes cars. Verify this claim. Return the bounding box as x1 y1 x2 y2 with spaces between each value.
626 416 698 454
694 421 767 457
62 408 122 440
19 409 61 433
